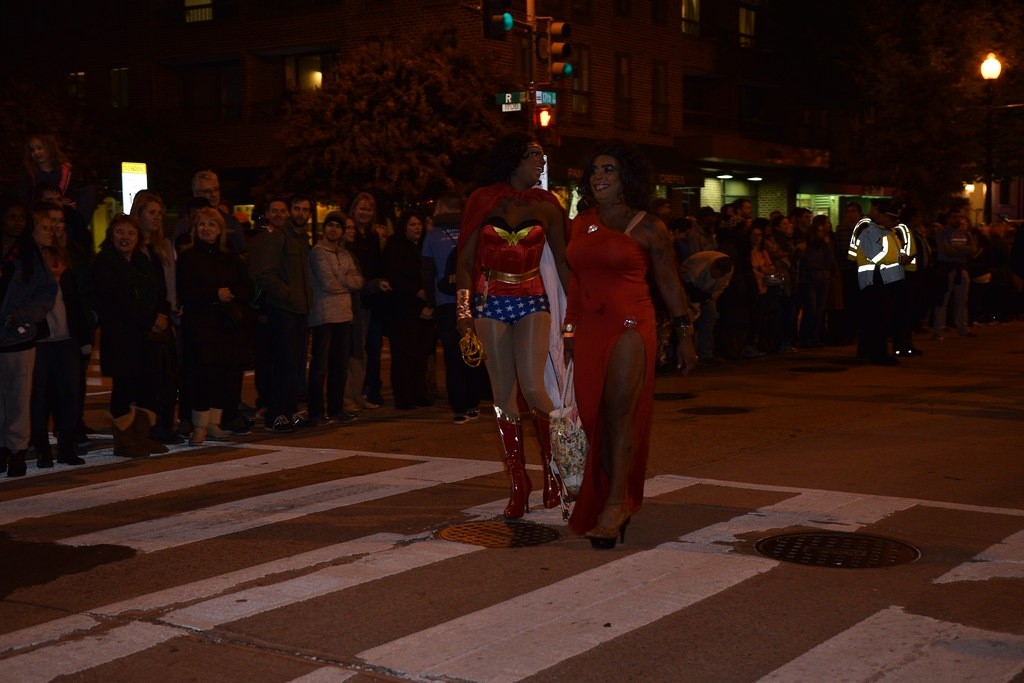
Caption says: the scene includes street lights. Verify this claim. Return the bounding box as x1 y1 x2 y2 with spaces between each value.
980 53 1001 227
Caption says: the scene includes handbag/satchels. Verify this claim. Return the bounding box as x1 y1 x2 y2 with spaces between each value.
0 307 36 348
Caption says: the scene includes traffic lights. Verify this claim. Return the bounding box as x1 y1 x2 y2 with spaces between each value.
548 22 572 81
534 107 556 127
483 0 513 41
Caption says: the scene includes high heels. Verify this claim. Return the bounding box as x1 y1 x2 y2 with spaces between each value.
586 503 631 550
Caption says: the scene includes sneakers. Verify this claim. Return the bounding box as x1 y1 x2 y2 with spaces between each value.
285 411 315 430
264 414 297 433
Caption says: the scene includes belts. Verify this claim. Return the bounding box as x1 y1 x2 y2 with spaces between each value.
480 265 541 285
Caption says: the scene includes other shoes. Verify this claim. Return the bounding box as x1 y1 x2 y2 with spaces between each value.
36 451 55 468
6 451 27 477
307 394 481 428
53 419 97 448
656 309 1024 376
0 446 7 473
220 412 256 436
56 448 85 466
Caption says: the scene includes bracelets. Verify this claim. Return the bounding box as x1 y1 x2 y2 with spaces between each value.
672 314 694 338
457 288 473 319
562 322 576 338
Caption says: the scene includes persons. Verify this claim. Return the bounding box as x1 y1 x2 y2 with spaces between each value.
561 136 698 550
456 131 570 518
653 194 1024 375
0 133 483 478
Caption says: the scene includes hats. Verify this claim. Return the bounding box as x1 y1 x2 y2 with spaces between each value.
870 198 894 208
322 210 348 231
879 206 900 218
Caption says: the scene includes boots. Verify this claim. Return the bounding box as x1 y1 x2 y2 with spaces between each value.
188 408 211 446
149 416 185 445
132 406 169 454
205 407 240 442
492 403 533 519
103 405 151 457
529 406 562 508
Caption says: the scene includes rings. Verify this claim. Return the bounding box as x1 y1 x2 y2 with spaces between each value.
696 356 698 358
